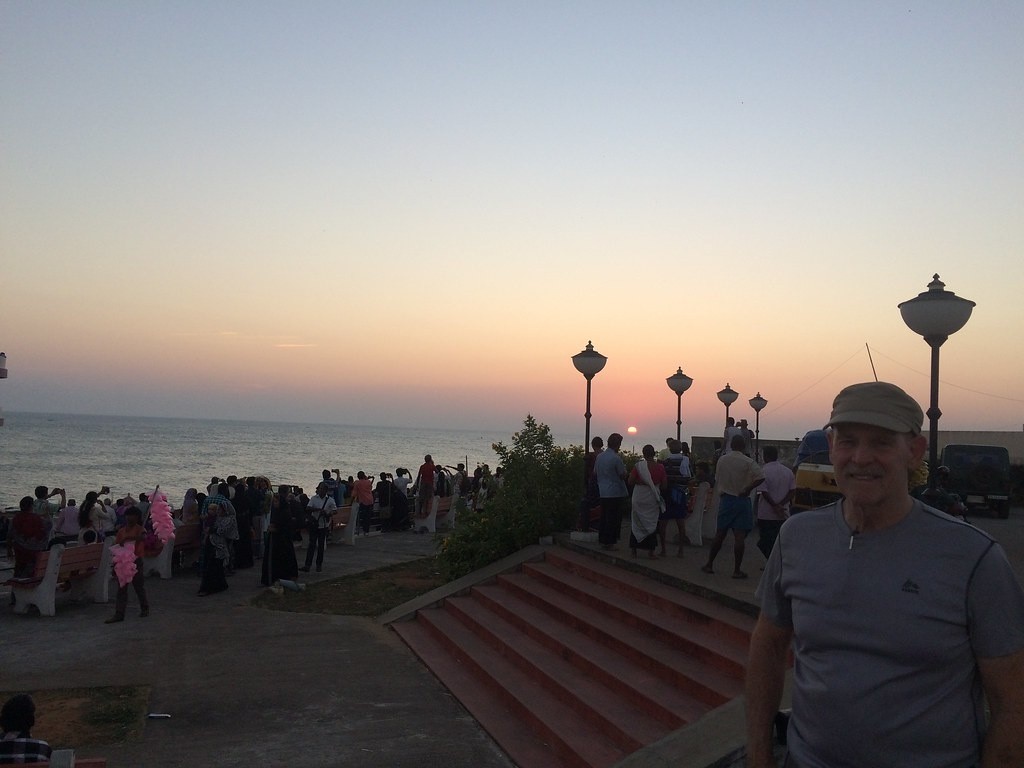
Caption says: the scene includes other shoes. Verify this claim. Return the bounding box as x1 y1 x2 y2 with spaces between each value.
731 571 747 578
196 591 208 596
138 607 150 617
601 543 619 550
298 566 310 572
701 564 715 574
104 612 124 624
316 566 321 572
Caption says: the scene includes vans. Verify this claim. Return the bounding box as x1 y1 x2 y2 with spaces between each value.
938 443 1012 520
788 429 845 515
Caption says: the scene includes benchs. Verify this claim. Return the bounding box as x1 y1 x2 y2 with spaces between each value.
3 536 116 617
302 503 360 548
664 478 717 546
142 522 200 579
415 493 458 534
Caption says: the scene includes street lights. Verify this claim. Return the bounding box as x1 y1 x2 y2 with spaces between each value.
570 340 609 531
716 383 739 429
748 392 768 464
896 273 977 509
665 366 694 441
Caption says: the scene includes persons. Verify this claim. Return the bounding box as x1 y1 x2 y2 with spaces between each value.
628 445 668 558
174 476 274 596
702 435 765 578
78 486 174 624
657 437 716 558
744 382 1023 768
708 418 755 484
0 694 53 765
593 433 629 551
412 455 466 518
467 465 503 515
341 471 374 535
921 466 967 521
0 486 79 605
756 447 796 571
261 469 348 592
376 468 412 532
582 436 604 532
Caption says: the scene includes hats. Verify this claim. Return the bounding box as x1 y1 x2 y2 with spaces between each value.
823 381 927 437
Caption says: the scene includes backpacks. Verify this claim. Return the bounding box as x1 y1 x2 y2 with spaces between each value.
458 470 471 487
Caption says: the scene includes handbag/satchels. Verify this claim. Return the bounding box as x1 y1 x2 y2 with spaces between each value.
303 514 319 535
379 506 392 519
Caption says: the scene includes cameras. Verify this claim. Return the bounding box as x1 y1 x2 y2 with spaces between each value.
56 489 62 493
102 486 109 493
219 478 223 482
332 469 338 472
402 469 407 474
